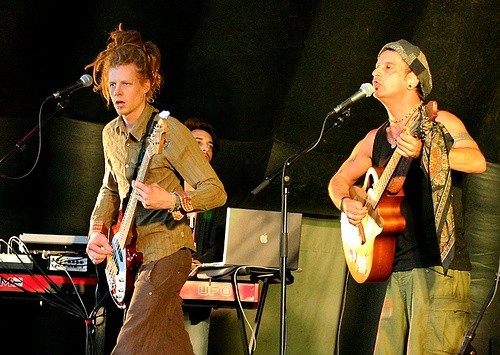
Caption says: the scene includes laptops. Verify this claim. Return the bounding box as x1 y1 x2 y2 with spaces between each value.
198 206 303 270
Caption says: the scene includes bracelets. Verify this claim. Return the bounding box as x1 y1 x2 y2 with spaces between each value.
170 195 181 211
340 197 350 211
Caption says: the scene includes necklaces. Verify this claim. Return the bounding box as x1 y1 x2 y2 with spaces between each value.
388 106 418 123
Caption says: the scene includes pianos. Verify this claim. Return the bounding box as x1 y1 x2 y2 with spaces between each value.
0 233 261 355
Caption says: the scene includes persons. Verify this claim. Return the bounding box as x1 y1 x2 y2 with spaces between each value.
85 30 227 355
185 119 213 355
328 39 487 355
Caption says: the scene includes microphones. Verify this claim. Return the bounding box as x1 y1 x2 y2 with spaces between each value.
330 82 375 114
48 74 93 100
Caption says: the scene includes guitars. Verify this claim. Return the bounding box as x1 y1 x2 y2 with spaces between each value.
340 99 438 284
104 109 171 308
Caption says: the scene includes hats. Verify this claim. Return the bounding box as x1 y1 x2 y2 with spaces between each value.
377 39 432 97
